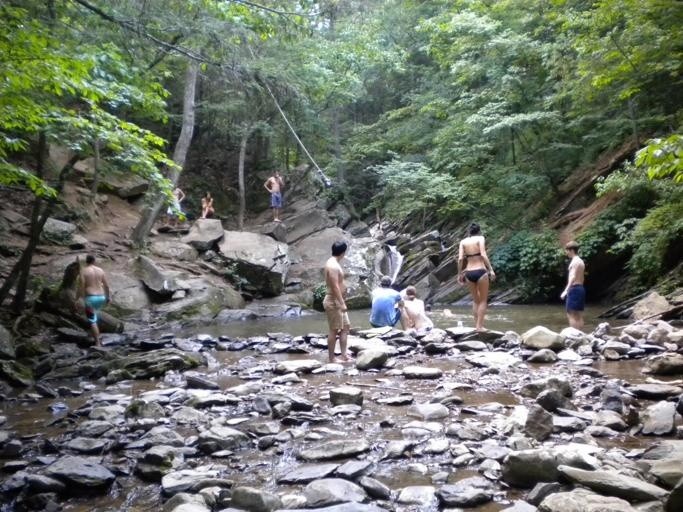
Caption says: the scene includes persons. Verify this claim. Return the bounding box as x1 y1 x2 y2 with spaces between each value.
368 275 402 328
320 240 354 364
455 222 496 334
71 254 109 347
400 285 434 331
198 189 215 219
262 170 284 224
558 240 586 331
166 181 185 224
